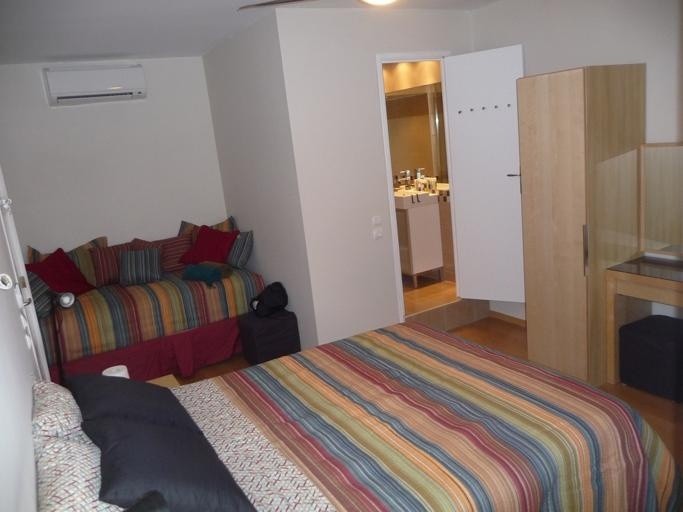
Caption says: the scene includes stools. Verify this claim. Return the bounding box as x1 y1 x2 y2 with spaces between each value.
235 310 300 367
617 313 681 407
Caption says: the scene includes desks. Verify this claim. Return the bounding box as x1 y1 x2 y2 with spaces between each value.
603 256 681 386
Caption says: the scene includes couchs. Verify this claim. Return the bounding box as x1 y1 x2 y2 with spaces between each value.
35 241 267 385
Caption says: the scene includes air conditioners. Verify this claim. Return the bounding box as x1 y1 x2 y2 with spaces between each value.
41 63 149 108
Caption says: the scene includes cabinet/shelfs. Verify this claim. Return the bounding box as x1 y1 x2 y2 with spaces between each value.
513 60 647 390
393 202 445 291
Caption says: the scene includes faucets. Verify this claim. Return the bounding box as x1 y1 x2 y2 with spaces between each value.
398 170 412 188
416 167 425 180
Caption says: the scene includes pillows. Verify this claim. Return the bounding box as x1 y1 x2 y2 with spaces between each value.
81 419 257 512
57 373 194 420
31 435 126 512
30 379 101 447
24 215 256 320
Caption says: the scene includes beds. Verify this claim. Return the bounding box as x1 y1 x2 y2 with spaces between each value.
30 318 650 512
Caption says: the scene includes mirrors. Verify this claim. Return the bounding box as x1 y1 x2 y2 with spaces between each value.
637 142 682 259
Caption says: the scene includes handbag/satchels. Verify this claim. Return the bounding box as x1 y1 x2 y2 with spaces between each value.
251 282 287 316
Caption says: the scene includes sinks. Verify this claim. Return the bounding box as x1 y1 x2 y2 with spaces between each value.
395 189 429 210
437 182 449 202
